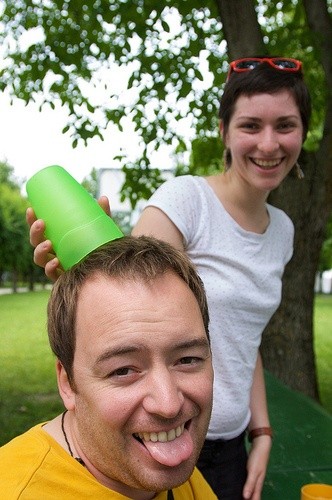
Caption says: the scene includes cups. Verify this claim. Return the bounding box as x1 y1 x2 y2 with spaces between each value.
27 164 126 272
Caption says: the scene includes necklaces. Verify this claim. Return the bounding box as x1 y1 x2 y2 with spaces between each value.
62 411 84 467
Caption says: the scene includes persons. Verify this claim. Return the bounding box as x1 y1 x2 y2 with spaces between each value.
26 55 310 499
1 236 216 499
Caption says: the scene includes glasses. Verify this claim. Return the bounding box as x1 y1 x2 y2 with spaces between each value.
225 58 302 83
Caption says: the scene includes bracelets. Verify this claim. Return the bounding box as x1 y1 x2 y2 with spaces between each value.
248 427 273 442
